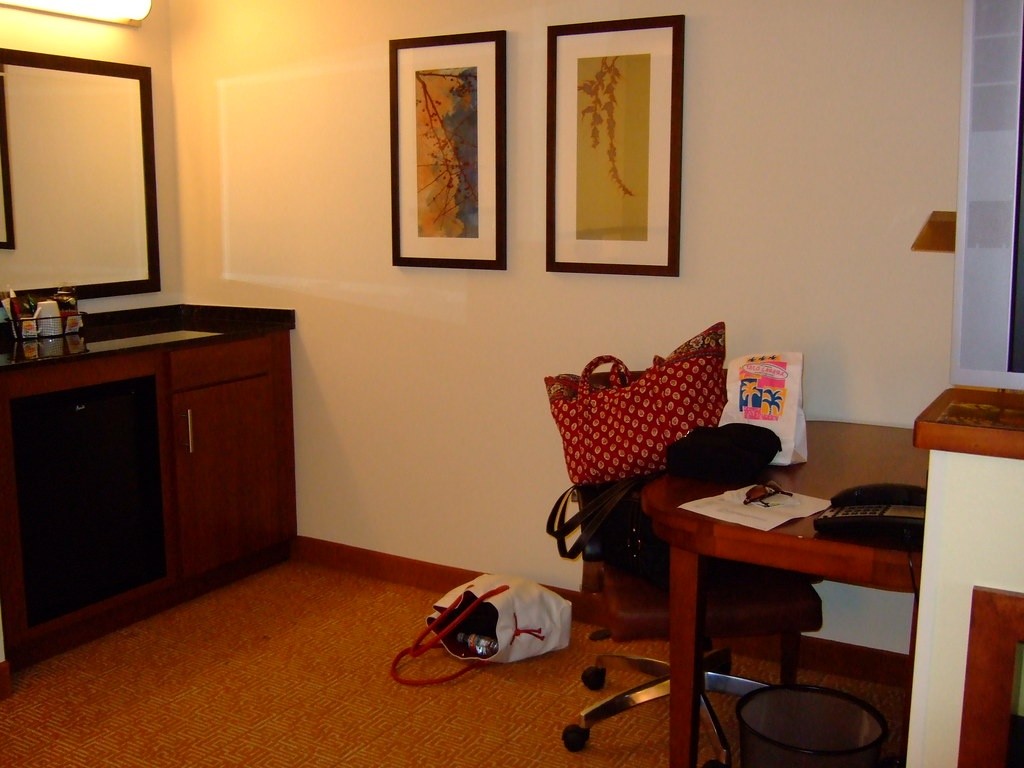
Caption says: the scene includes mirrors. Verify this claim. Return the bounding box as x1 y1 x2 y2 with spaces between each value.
0 47 161 307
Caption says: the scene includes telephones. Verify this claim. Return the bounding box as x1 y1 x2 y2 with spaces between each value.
813 482 927 538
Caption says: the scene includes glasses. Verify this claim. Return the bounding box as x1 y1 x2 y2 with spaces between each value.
743 479 792 507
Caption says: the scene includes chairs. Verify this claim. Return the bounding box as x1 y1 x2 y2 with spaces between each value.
562 560 823 768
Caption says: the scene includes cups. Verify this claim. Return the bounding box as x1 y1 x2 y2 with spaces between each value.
18 300 65 339
20 337 64 361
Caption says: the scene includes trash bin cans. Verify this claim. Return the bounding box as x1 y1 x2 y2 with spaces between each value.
735 683 890 768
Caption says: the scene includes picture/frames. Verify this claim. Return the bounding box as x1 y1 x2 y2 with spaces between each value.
387 31 508 272
544 14 686 277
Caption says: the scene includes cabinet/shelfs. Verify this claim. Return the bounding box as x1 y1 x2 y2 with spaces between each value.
0 330 296 673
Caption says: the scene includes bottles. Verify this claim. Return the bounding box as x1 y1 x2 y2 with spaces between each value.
456 631 498 655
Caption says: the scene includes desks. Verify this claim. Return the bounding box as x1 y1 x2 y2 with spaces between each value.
640 420 928 768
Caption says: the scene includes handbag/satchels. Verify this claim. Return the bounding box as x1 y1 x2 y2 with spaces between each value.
543 321 729 486
392 573 573 686
718 351 807 466
546 478 670 562
667 423 782 484
